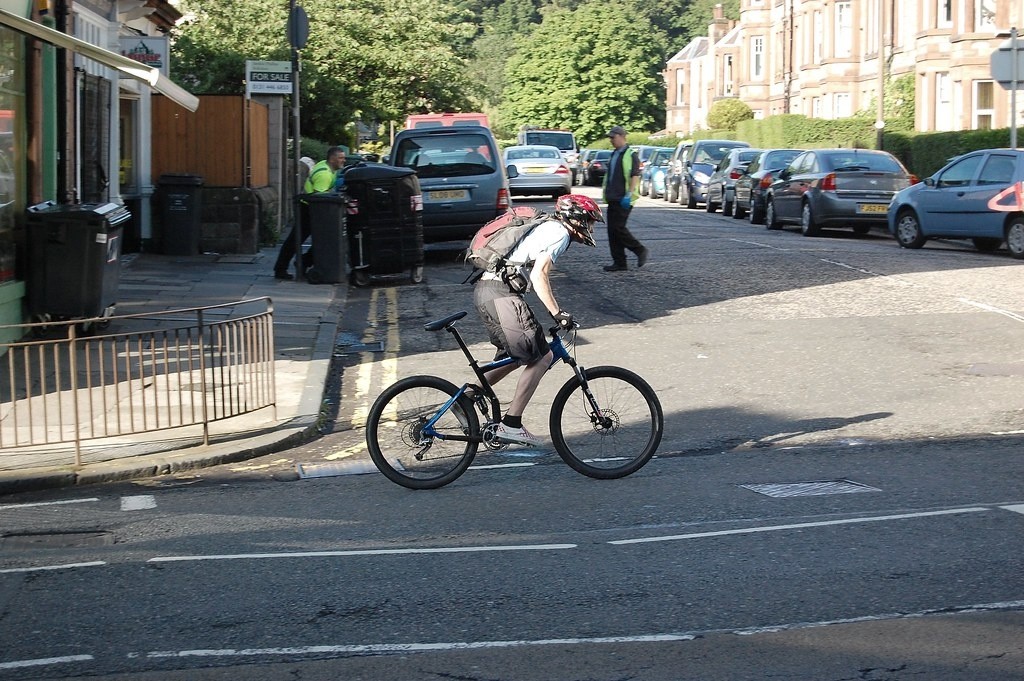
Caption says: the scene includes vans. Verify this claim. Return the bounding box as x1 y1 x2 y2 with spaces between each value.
384 123 517 241
405 112 493 163
0 109 15 149
522 130 579 184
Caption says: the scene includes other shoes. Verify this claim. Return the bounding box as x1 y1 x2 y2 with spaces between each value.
603 261 627 270
274 272 293 279
638 248 648 267
295 253 307 276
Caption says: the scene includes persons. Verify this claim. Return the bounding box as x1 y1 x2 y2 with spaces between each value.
602 127 649 271
272 146 357 280
448 194 605 447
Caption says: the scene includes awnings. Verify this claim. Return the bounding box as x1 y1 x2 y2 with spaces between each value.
0 8 199 113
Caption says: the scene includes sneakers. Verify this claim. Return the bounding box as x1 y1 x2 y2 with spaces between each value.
495 422 539 446
449 400 470 433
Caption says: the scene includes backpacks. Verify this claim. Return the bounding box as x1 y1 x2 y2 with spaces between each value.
455 206 573 294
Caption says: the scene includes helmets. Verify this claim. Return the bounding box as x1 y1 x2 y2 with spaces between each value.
554 194 606 248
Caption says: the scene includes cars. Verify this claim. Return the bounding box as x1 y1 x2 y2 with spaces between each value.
503 146 574 199
575 144 656 187
1 151 17 228
887 148 1024 258
411 151 492 162
641 148 675 199
732 148 805 225
664 141 711 205
678 141 752 207
765 147 920 235
705 147 767 216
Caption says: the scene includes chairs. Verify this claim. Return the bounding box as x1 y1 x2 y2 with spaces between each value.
464 152 488 163
539 150 555 158
412 153 430 167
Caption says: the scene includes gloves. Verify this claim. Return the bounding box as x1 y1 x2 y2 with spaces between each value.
554 310 573 330
621 192 634 210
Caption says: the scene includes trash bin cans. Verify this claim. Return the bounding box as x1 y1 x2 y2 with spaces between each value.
24 201 132 336
158 172 202 257
337 162 425 285
293 194 347 283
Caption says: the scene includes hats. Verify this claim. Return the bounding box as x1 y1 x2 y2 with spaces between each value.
605 126 626 137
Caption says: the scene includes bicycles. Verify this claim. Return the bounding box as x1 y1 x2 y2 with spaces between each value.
366 308 663 491
